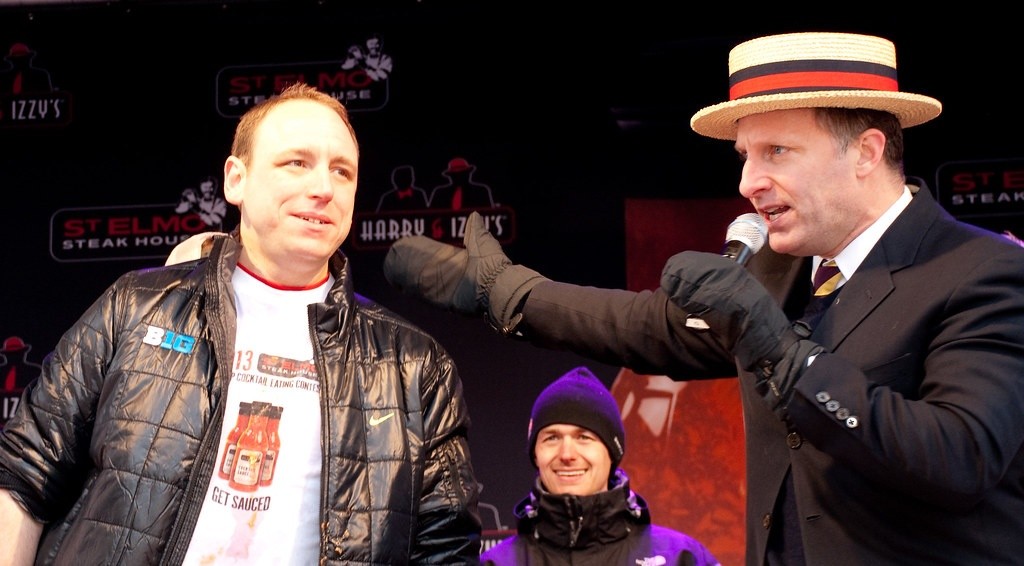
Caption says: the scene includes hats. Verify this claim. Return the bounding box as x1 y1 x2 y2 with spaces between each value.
690 32 942 142
164 231 233 264
527 367 625 478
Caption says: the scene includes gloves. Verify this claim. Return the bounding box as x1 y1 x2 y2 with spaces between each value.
383 211 553 341
660 251 828 421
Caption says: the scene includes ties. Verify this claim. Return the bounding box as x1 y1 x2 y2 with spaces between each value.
811 259 843 297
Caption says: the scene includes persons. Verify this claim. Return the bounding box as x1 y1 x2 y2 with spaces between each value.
0 84 480 566
383 32 1024 566
165 231 234 267
481 366 723 566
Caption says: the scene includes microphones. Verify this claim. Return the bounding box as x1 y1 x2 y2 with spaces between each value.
687 212 770 331
